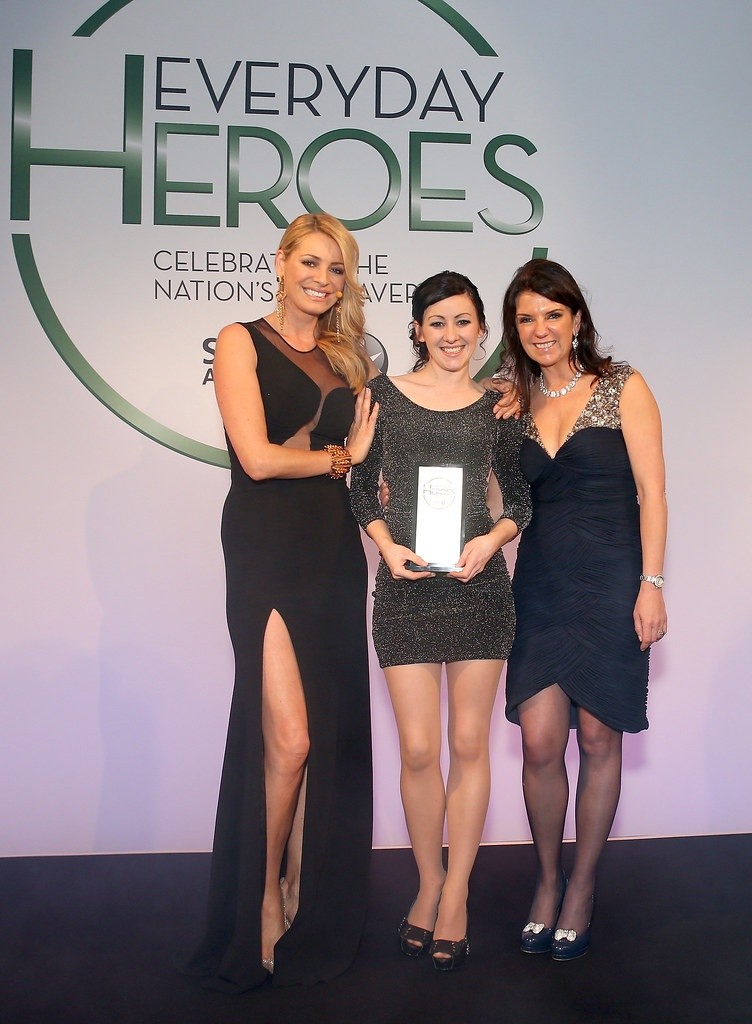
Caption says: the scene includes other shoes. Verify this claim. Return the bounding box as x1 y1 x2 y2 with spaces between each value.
397 896 438 960
429 915 469 973
261 877 290 974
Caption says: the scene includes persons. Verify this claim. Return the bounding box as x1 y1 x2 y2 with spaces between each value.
351 271 532 971
377 256 667 963
191 213 523 993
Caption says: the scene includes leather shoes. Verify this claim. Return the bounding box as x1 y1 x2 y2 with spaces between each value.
551 886 595 961
520 878 568 953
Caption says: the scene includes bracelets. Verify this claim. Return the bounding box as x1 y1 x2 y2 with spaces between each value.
324 443 352 480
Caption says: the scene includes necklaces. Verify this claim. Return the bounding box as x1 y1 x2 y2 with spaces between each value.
539 362 587 398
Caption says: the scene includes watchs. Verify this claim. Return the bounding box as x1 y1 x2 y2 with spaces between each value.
640 574 666 588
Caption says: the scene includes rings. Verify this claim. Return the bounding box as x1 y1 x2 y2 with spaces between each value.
517 399 522 403
658 631 664 635
